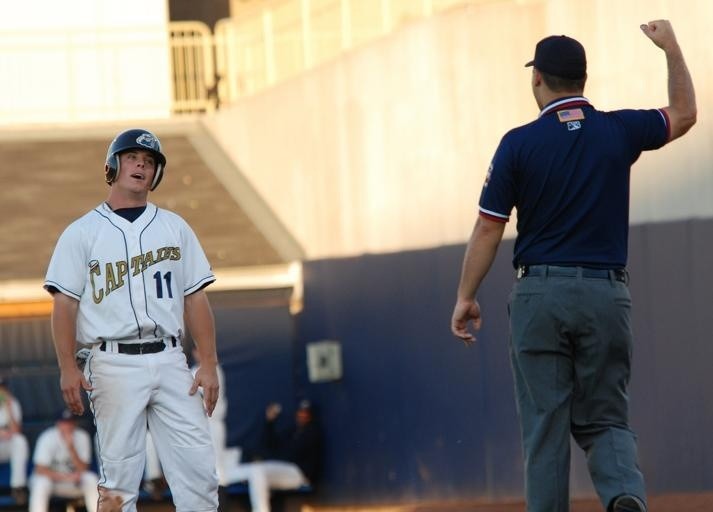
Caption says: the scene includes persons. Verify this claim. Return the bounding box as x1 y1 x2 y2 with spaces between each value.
144 431 165 502
451 20 697 512
190 344 225 484
28 408 98 511
42 129 219 512
0 371 29 506
256 397 328 511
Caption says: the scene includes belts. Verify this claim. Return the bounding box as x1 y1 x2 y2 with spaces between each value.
517 266 625 282
99 338 176 355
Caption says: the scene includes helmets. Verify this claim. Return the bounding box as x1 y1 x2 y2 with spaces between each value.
104 129 166 192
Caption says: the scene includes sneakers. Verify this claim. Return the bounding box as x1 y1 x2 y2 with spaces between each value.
613 494 646 512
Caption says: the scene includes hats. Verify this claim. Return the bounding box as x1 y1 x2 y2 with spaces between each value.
525 35 586 80
